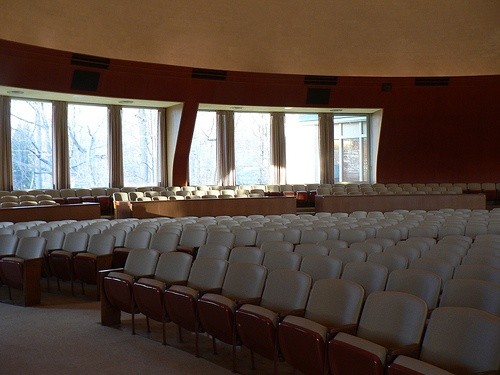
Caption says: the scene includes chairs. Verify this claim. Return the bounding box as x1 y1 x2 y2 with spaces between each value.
0 183 500 375
